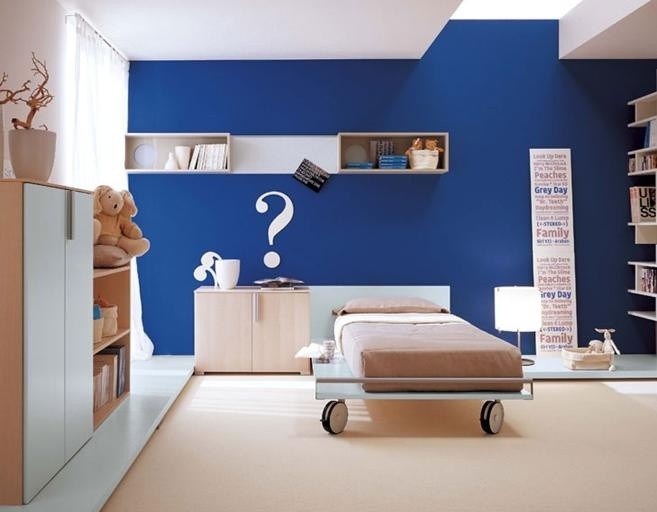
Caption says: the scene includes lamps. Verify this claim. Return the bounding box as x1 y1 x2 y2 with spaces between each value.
493 286 542 367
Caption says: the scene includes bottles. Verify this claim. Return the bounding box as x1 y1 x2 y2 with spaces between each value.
164 152 178 170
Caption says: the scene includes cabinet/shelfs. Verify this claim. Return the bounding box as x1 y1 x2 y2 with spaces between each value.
625 90 656 321
192 284 311 378
123 132 230 175
336 132 449 175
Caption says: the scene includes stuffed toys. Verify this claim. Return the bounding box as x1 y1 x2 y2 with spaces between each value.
403 137 424 156
593 327 620 371
422 137 444 152
91 185 150 270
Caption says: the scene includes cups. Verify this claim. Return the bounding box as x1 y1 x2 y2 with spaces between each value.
174 146 191 171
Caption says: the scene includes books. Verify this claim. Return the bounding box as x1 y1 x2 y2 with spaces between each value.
91 343 127 411
187 143 228 170
293 340 341 360
638 267 656 294
345 153 408 170
627 152 656 173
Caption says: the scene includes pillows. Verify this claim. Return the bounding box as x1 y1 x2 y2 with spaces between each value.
330 297 451 315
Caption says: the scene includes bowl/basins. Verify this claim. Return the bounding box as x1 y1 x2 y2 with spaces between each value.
407 150 438 170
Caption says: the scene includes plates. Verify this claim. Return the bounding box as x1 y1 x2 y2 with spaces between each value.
345 144 368 165
345 163 376 169
377 154 408 169
252 277 305 287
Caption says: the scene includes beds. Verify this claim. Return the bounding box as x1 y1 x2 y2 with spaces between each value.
331 304 523 393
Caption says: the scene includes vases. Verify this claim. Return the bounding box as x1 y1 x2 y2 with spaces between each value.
8 127 58 183
